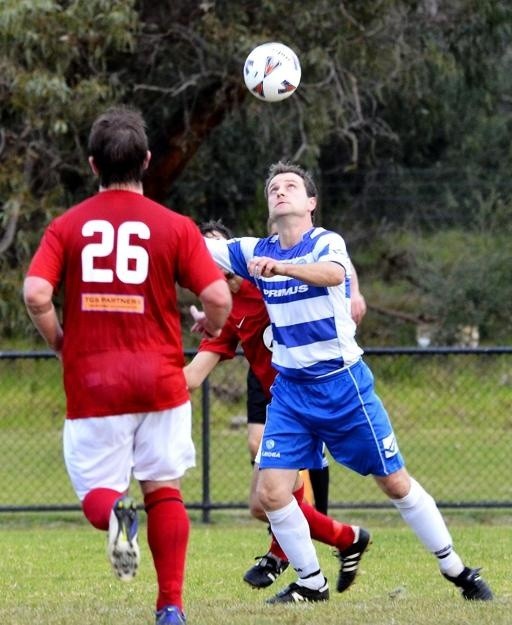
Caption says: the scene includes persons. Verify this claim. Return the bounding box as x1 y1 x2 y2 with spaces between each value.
21 106 232 624
180 220 370 597
195 158 498 604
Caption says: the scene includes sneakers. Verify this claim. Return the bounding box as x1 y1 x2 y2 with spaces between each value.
443 564 491 600
269 576 329 603
243 551 290 588
107 496 141 581
336 524 370 593
156 606 186 625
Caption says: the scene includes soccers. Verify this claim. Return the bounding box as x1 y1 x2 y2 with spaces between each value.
244 43 301 102
